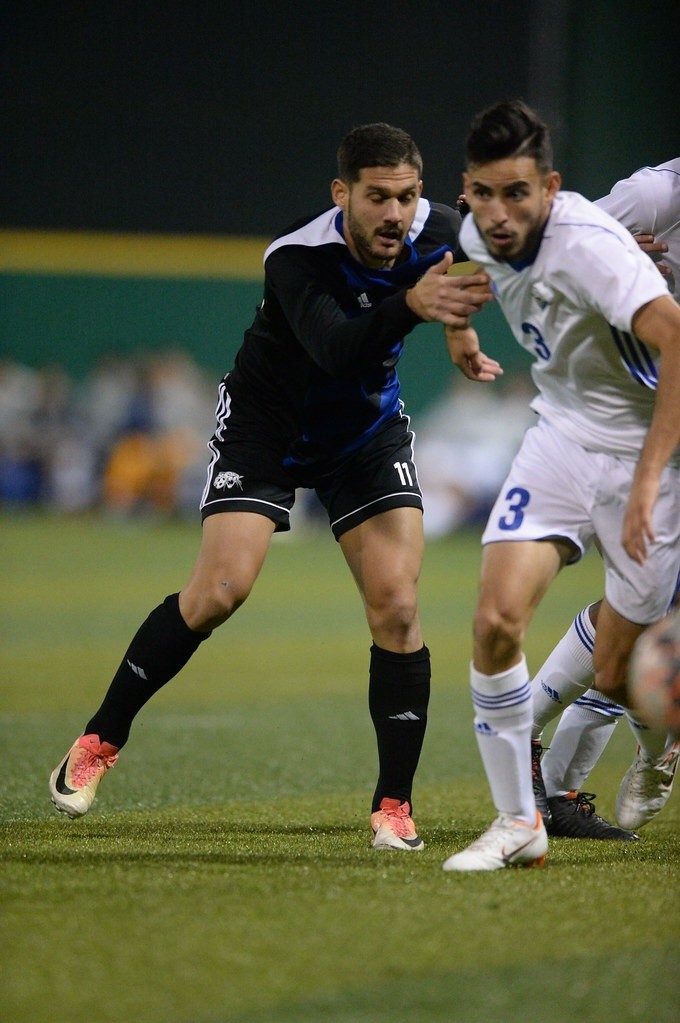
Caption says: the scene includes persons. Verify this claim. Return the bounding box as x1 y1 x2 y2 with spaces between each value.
51 100 680 869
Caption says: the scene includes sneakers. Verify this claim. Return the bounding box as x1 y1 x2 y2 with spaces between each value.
546 792 641 842
615 740 680 830
531 740 552 819
370 797 424 851
442 810 549 870
49 732 119 820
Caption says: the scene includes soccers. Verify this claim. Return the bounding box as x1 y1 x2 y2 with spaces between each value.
627 609 680 733
